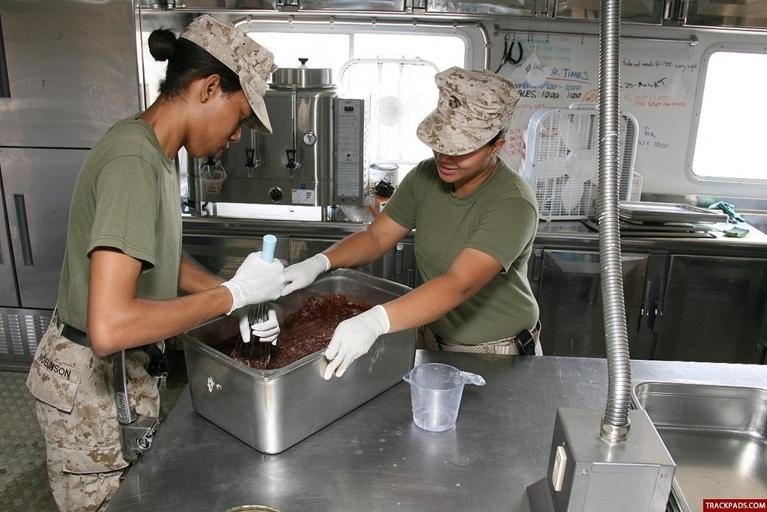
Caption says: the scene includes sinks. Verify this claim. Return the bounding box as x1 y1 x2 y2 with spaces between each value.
627 381 766 511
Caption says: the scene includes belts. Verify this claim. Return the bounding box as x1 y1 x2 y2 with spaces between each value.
436 319 541 355
57 313 90 348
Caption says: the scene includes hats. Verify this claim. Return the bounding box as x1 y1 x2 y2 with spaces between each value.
417 66 521 156
181 15 278 135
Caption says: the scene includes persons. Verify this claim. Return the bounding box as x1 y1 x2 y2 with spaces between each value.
26 14 287 512
281 67 544 380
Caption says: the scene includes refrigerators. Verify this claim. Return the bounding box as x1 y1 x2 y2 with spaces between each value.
0 3 186 316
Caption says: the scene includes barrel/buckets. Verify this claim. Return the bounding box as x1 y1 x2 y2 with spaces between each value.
193 89 338 205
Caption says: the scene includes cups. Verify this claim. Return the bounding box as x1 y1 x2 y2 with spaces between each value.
401 362 486 434
199 170 226 202
368 162 400 191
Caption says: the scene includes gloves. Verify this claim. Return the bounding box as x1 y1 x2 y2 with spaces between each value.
324 304 389 381
282 253 330 297
219 250 285 316
232 302 281 345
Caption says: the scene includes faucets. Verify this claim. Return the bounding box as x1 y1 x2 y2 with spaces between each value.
285 149 299 180
245 148 256 179
205 157 216 177
